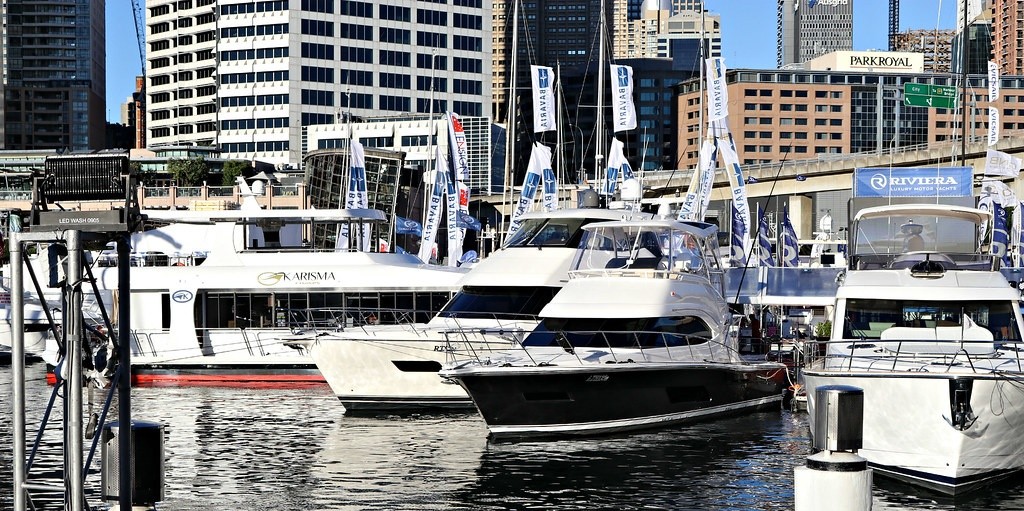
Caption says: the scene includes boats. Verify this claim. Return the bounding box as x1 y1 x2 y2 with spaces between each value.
709 236 849 355
2 244 113 326
803 206 1024 500
765 337 820 370
43 205 473 391
727 314 752 354
284 209 664 423
0 267 97 364
737 329 770 363
438 219 796 442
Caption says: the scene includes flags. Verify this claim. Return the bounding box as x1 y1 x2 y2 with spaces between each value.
730 202 799 268
531 64 556 133
665 58 751 249
984 149 1022 178
988 106 999 147
374 238 389 252
988 61 999 103
418 111 481 267
504 143 559 243
602 138 634 194
611 64 637 133
396 217 421 237
980 177 1024 268
338 139 372 252
